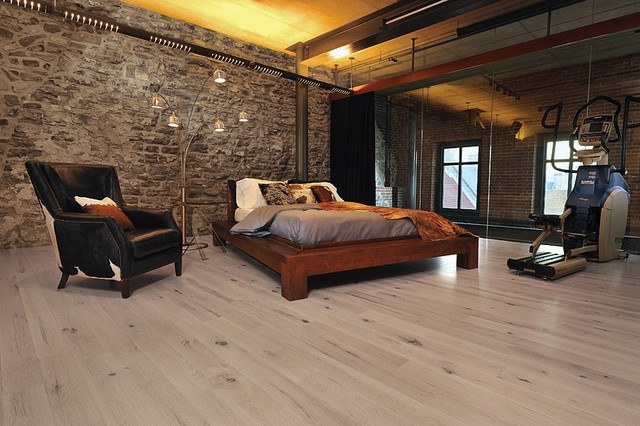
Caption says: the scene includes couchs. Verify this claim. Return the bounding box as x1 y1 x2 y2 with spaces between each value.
22 157 185 299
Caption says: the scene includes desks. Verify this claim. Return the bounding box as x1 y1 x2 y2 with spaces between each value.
169 196 230 261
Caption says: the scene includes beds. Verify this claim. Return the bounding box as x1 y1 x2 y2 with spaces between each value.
210 177 480 301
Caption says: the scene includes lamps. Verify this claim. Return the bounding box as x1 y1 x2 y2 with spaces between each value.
150 66 248 249
